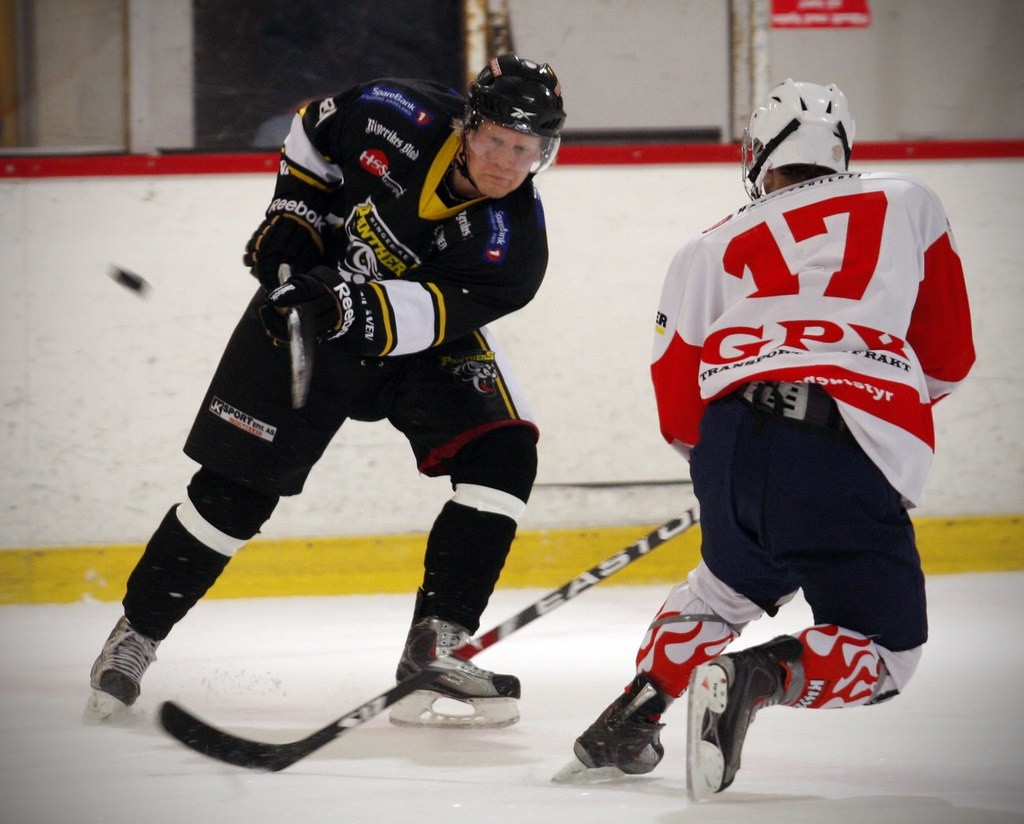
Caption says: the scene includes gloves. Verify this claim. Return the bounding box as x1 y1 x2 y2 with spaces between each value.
257 277 398 358
242 209 336 285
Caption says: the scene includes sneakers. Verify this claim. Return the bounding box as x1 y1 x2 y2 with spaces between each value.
387 586 522 729
686 634 805 801
85 615 161 725
572 673 666 774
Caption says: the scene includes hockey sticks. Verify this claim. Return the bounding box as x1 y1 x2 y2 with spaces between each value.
156 499 701 771
287 305 317 412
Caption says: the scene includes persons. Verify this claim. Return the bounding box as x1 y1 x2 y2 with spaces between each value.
552 78 977 802
85 53 566 729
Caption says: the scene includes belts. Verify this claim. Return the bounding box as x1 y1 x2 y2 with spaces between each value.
734 382 846 431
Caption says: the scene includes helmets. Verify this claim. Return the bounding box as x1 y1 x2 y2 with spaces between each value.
464 52 567 138
740 77 857 201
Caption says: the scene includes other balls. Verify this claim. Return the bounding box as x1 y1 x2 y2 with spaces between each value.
114 267 145 293
